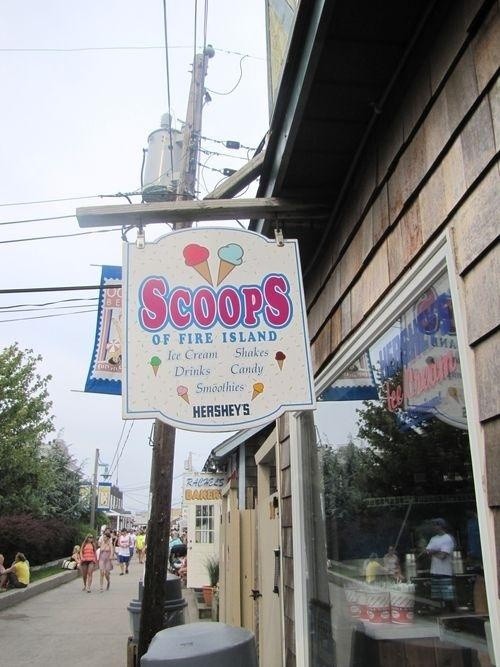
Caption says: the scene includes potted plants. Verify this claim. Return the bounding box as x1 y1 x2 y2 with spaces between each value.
202 552 220 606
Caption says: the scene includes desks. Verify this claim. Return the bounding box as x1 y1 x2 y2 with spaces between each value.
356 614 442 641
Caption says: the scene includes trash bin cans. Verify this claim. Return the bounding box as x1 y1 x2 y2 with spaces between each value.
140 621 259 667
127 572 188 640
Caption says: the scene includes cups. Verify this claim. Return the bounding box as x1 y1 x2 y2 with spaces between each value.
345 582 417 624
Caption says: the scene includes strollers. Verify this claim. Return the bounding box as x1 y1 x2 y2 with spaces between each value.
169 543 188 574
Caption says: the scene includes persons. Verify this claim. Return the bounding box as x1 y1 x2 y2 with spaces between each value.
363 536 432 585
1 551 30 592
465 504 483 610
420 518 457 613
70 524 189 593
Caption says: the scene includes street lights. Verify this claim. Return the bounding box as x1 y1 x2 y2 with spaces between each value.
89 463 113 529
